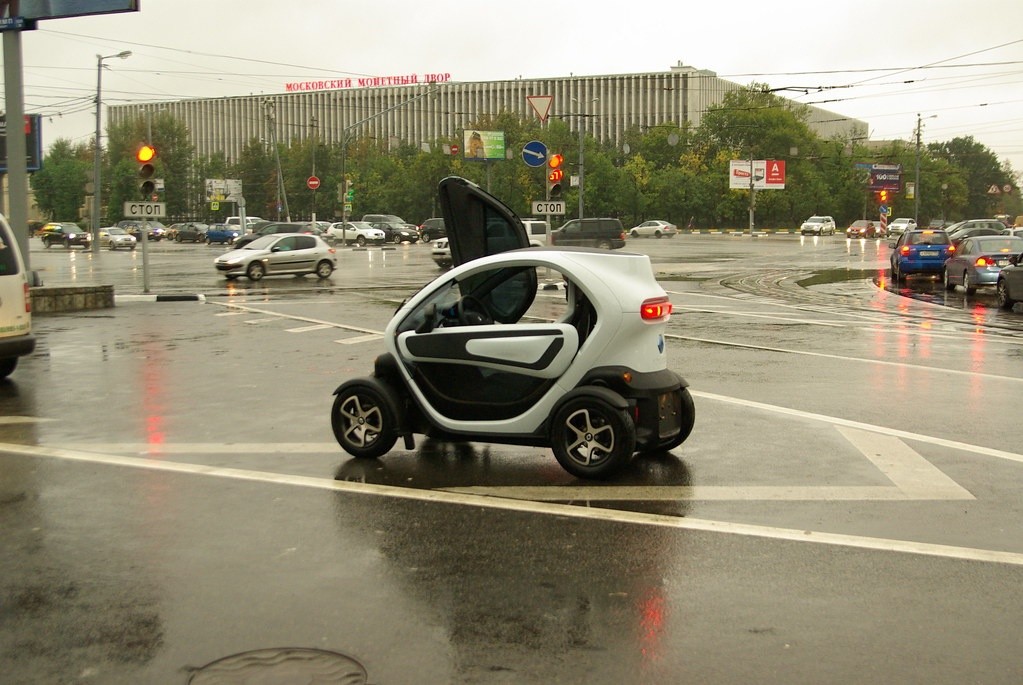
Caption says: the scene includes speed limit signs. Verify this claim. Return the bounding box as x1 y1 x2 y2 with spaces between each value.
1003 184 1012 192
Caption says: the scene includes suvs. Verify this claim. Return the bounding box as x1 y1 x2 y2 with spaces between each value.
40 222 92 248
420 216 549 268
361 214 419 231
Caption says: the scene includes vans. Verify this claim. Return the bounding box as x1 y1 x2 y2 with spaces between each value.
946 218 1007 235
117 220 168 241
551 216 626 250
225 216 263 224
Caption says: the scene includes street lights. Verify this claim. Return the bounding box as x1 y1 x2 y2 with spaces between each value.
915 114 939 225
571 97 601 219
92 51 132 253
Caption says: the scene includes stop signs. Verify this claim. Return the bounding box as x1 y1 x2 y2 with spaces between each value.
307 176 320 189
451 145 458 154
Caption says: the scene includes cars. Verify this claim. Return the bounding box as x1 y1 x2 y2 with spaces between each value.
629 220 678 238
886 217 918 237
170 222 241 246
800 215 836 236
214 220 421 283
99 227 137 250
846 219 882 238
926 219 946 230
329 244 696 482
0 212 36 377
888 227 1023 308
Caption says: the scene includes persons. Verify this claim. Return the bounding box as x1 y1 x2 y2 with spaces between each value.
465 132 486 158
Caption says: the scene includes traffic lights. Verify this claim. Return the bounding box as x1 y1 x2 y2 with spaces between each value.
345 179 355 197
548 153 564 185
880 190 889 204
135 145 156 195
